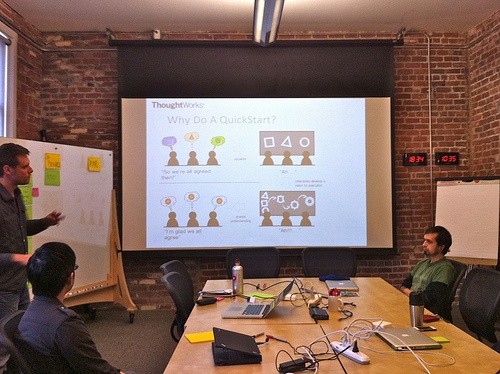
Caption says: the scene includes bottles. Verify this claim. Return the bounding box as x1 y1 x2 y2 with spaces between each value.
232 259 243 294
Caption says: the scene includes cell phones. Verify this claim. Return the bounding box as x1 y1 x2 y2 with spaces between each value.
413 326 437 331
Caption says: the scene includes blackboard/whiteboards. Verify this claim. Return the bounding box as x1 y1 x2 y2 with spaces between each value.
432 176 499 267
0 135 119 301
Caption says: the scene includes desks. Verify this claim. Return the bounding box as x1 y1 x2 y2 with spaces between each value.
161 325 347 374
321 325 500 374
183 278 316 326
295 276 434 325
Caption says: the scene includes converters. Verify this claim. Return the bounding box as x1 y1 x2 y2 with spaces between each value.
279 359 306 374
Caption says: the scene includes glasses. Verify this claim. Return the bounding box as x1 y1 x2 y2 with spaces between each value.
71 264 78 273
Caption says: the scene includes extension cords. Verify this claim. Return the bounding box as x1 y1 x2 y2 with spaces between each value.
330 341 371 365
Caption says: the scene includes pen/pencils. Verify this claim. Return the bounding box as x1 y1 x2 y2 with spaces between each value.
266 334 288 343
253 332 264 338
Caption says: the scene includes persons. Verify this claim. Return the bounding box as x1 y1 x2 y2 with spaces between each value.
399 226 456 319
15 242 136 374
0 143 65 318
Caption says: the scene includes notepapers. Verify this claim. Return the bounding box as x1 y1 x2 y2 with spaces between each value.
431 336 450 343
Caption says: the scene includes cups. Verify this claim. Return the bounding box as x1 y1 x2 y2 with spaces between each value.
328 296 344 312
410 292 425 328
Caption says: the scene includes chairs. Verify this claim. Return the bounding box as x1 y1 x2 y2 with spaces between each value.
160 258 194 344
0 311 34 374
302 248 357 277
447 259 500 346
227 247 279 279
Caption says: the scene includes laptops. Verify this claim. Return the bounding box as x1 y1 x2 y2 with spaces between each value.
212 327 262 366
374 327 443 351
221 279 296 319
325 280 359 292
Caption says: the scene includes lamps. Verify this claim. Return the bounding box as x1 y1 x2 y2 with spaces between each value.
253 0 285 48
